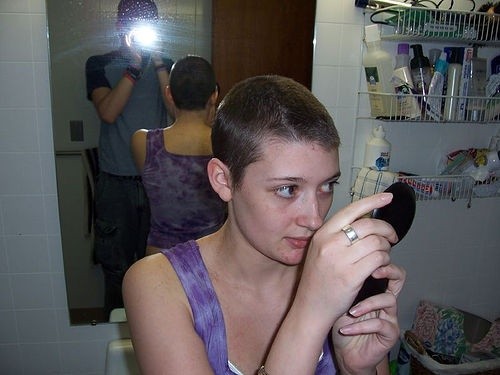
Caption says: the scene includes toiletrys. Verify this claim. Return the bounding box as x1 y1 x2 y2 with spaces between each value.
399 174 440 199
363 23 500 124
363 125 390 171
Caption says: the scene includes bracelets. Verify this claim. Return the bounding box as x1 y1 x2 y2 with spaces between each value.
258 366 267 375
124 65 139 83
155 64 166 72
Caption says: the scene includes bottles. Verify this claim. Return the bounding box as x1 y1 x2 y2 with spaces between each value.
394 43 410 67
363 24 398 117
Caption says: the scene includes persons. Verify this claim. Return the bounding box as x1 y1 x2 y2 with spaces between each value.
122 77 406 375
131 55 228 257
86 0 176 321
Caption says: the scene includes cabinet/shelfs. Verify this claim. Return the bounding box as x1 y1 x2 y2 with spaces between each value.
350 6 500 208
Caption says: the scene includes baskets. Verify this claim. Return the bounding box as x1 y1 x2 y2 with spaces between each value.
398 325 500 375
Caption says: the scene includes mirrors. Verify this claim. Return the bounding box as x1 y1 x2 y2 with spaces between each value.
45 0 317 327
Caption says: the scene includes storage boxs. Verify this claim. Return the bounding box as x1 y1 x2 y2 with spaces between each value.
398 329 500 375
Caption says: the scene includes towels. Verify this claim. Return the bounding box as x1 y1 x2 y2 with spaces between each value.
81 147 101 270
352 166 400 204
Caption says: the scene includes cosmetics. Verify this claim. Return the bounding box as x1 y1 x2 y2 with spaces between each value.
363 24 500 121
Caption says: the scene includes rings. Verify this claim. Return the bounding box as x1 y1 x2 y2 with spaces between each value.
342 224 359 245
